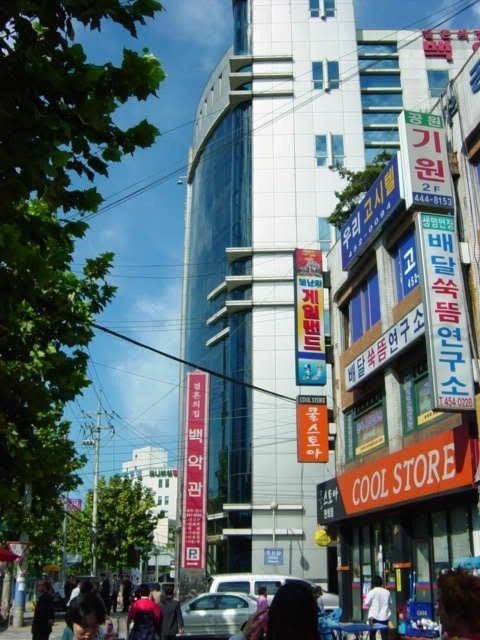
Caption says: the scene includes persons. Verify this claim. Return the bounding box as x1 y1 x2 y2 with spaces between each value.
30 580 56 640
121 575 132 613
60 573 125 640
434 565 480 639
157 584 185 640
247 585 268 638
226 580 322 639
364 574 393 640
148 583 164 603
125 584 161 640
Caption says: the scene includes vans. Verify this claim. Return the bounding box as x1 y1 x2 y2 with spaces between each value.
208 573 340 612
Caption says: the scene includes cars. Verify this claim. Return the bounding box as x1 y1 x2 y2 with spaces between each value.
179 591 261 636
